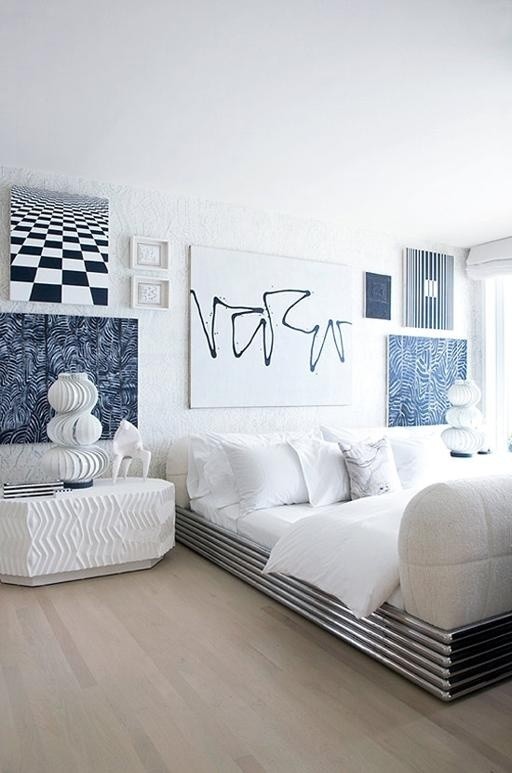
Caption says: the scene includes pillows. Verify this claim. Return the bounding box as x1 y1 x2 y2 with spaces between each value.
186 424 425 517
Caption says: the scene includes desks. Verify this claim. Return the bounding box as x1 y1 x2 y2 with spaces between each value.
0 476 178 587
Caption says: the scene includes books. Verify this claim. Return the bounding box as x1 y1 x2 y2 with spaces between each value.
3 482 66 499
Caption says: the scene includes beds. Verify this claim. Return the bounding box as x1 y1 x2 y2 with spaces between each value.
163 436 512 705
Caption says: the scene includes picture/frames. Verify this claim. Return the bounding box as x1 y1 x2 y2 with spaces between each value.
131 235 171 311
